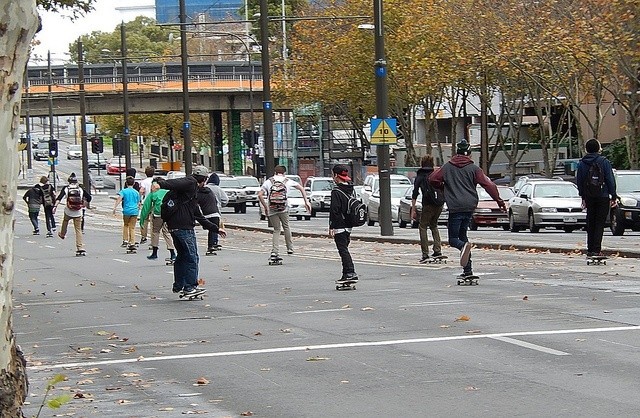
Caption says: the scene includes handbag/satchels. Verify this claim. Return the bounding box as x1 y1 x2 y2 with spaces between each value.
28 204 40 213
424 173 445 206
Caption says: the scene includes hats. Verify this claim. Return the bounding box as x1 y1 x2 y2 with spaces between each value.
585 138 601 153
457 139 471 156
68 172 77 183
194 164 210 178
332 164 351 182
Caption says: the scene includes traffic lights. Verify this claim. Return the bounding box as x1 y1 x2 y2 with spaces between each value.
49 140 58 157
113 139 126 156
92 137 103 154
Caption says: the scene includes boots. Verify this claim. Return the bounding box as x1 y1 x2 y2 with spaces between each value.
147 246 158 260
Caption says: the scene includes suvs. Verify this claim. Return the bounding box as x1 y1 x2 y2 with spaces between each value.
514 175 565 190
207 173 247 214
611 167 640 237
304 175 334 217
33 143 50 161
363 173 413 204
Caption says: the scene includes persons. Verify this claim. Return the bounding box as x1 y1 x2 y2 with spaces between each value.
23 184 44 235
576 138 618 258
411 155 445 263
113 166 227 299
430 139 507 278
40 176 56 238
258 165 312 262
52 172 92 252
329 165 367 286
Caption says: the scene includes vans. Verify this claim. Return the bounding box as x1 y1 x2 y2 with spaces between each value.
272 174 303 186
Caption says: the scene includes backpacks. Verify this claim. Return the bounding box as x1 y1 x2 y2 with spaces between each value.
333 187 368 227
584 156 607 197
161 191 195 223
40 185 53 209
66 185 83 211
269 177 288 211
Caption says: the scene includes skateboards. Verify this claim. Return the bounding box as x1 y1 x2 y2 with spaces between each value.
586 255 609 266
213 245 222 251
184 289 206 300
33 232 40 235
75 250 86 256
126 248 137 254
457 275 480 286
165 258 177 265
336 280 358 290
206 248 218 256
46 234 53 238
431 255 448 264
268 257 283 265
148 244 159 250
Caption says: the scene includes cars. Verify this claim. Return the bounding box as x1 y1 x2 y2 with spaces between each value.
65 145 83 160
399 185 450 229
366 185 411 226
507 181 588 233
468 184 516 231
259 184 312 220
236 176 262 208
106 157 127 175
88 153 108 170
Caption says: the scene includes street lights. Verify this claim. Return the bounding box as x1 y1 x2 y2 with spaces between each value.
357 21 392 235
167 33 257 180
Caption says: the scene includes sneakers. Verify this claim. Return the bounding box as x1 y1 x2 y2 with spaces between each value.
460 242 471 267
140 237 147 244
271 252 283 261
419 257 431 264
335 273 359 284
57 232 64 240
184 289 206 298
587 251 607 259
431 252 442 259
